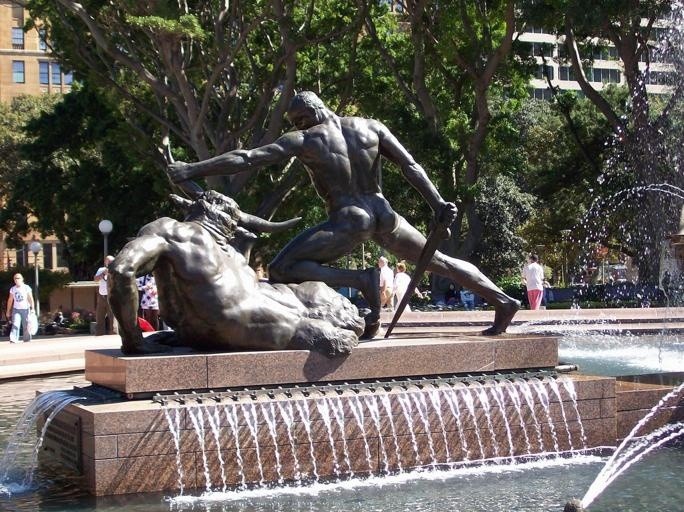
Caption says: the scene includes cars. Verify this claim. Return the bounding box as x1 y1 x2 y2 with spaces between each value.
576 261 630 284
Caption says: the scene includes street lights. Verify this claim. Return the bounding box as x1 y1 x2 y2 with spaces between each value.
558 225 574 287
97 219 114 277
27 240 41 316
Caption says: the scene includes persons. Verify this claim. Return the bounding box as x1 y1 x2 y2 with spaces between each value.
166 89 522 343
445 281 458 309
5 271 35 345
392 260 425 313
135 267 167 331
94 254 114 334
458 285 475 311
40 303 66 326
520 254 545 310
376 255 395 312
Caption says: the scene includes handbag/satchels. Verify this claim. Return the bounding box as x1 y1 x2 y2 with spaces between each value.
26 311 39 336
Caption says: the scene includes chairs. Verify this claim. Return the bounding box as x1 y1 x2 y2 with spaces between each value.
431 278 655 310
